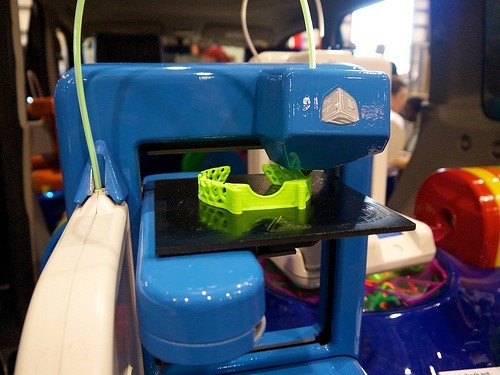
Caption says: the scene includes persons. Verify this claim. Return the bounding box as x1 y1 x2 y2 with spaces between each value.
387 78 413 170
367 45 390 59
